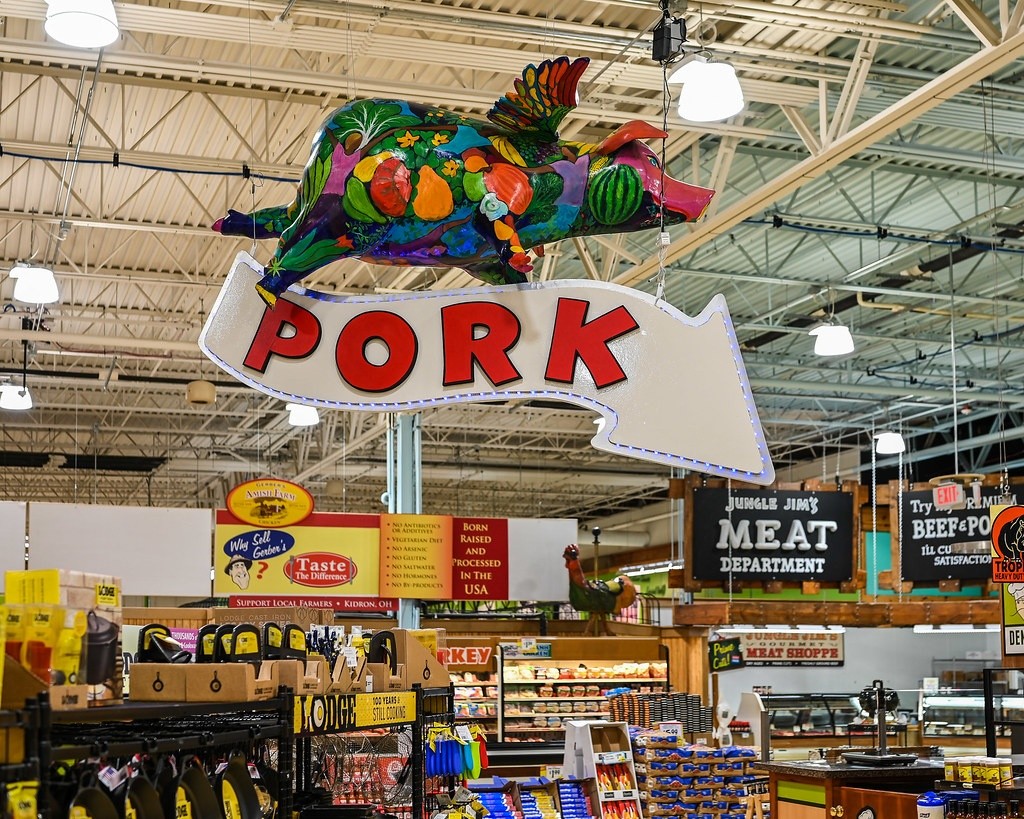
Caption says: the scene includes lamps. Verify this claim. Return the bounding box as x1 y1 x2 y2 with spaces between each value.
912 624 1002 633
713 625 848 635
0 0 908 458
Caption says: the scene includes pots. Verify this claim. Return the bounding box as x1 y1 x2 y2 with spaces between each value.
150 632 194 663
45 748 281 819
77 609 118 687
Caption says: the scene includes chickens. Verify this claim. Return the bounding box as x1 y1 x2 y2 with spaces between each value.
562 543 638 636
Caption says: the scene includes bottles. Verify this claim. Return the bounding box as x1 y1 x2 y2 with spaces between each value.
330 751 386 804
945 797 1024 819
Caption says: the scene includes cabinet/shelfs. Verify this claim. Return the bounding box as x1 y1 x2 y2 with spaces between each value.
2 608 1023 819
